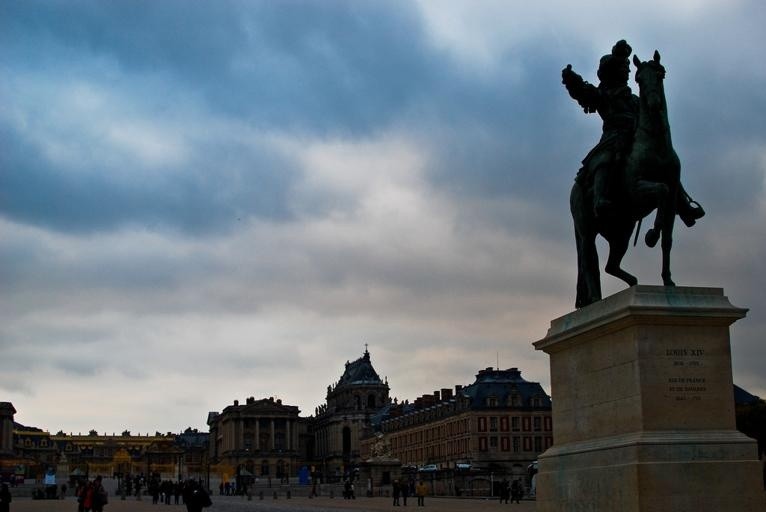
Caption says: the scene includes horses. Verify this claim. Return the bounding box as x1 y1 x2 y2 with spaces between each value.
569 49 685 310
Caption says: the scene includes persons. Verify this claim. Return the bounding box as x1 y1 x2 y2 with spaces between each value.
500 477 524 504
561 39 639 214
344 480 355 499
392 479 428 506
0 467 236 512
